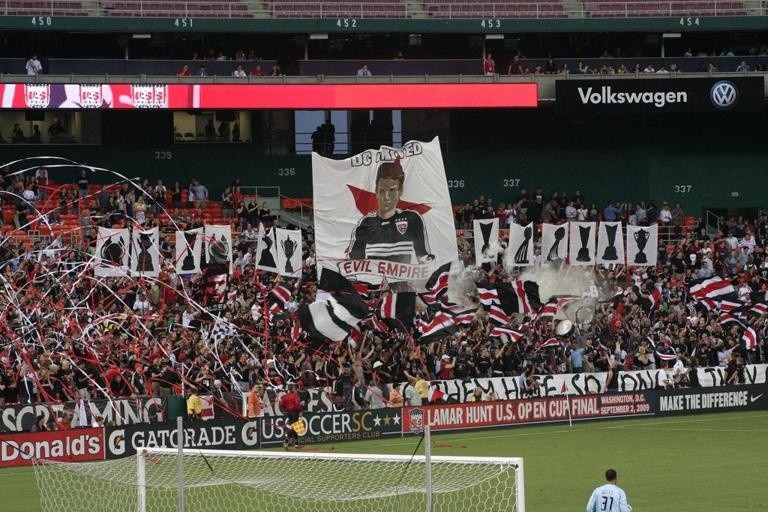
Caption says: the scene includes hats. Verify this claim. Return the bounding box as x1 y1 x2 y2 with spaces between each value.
0 219 467 389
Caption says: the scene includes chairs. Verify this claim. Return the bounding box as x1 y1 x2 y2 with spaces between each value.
1 0 747 19
0 182 700 255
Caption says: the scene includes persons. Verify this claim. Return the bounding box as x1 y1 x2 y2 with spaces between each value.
205 120 241 141
482 43 763 76
358 65 373 76
587 468 632 512
2 164 767 432
310 119 334 156
26 55 42 75
177 47 285 78
279 384 300 450
394 51 403 59
12 118 67 144
